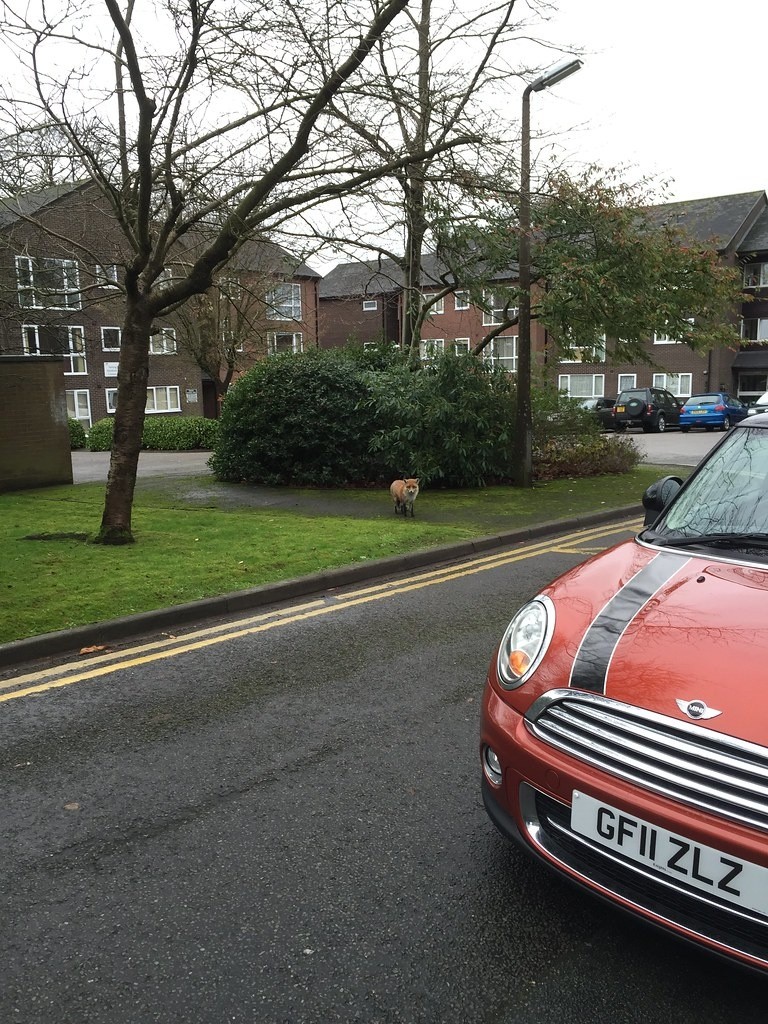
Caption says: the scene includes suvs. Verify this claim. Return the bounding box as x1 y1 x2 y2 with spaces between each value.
612 387 685 433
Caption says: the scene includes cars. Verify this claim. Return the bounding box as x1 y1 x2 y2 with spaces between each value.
478 413 768 975
746 392 768 417
678 391 748 433
579 397 616 429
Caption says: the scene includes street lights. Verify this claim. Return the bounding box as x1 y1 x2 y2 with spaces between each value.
518 60 586 488
